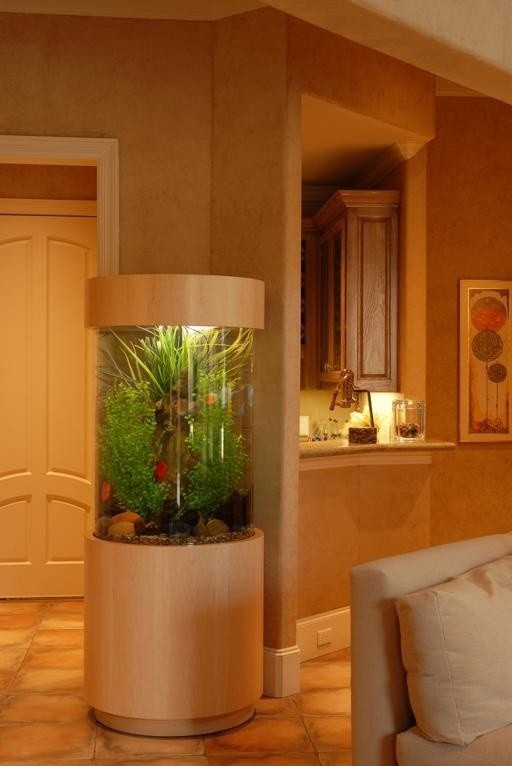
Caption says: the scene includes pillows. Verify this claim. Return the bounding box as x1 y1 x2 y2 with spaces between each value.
394 554 512 747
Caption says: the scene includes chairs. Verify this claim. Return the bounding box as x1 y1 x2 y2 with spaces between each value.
348 533 512 766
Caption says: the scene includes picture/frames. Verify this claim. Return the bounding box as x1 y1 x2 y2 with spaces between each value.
457 279 511 444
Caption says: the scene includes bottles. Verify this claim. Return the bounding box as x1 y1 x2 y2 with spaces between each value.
397 399 423 442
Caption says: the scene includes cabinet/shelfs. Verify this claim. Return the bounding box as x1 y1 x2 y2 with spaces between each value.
316 210 349 390
